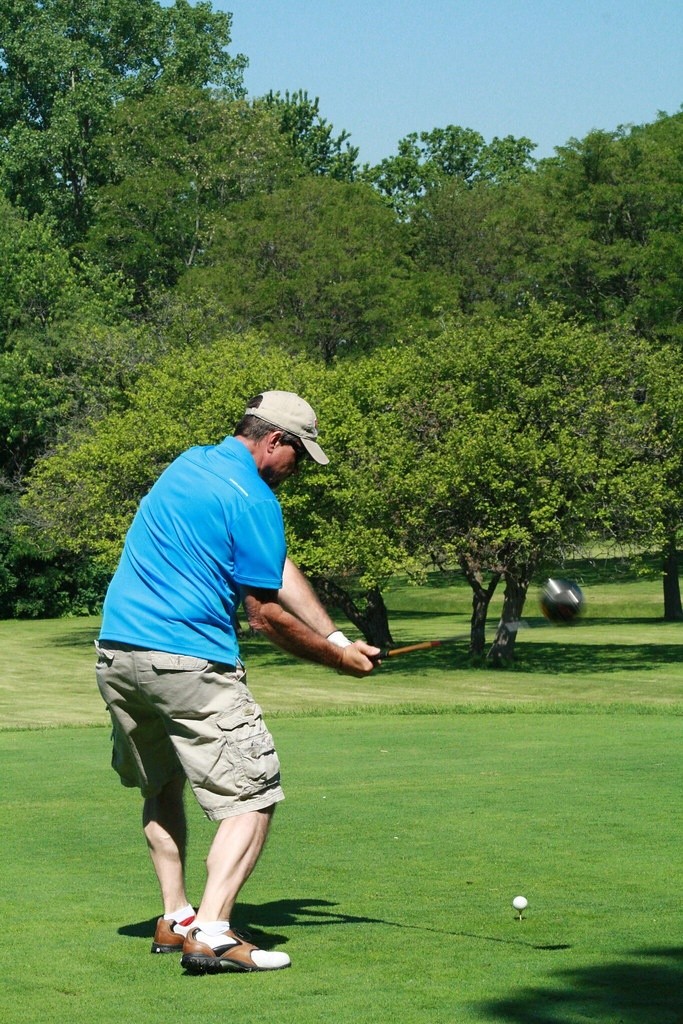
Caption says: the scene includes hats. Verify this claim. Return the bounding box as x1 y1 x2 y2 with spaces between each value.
245 390 331 465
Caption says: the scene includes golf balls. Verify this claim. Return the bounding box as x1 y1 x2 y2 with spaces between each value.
512 896 529 911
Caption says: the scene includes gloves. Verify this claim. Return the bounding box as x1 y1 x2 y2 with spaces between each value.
326 630 354 675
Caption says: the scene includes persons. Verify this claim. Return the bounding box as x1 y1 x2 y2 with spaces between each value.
93 391 382 971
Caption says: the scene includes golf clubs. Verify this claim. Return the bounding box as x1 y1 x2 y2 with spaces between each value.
335 577 585 676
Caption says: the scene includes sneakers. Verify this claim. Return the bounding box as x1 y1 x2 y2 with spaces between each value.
180 925 291 974
150 915 254 954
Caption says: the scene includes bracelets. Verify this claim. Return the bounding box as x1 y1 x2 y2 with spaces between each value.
325 630 355 648
